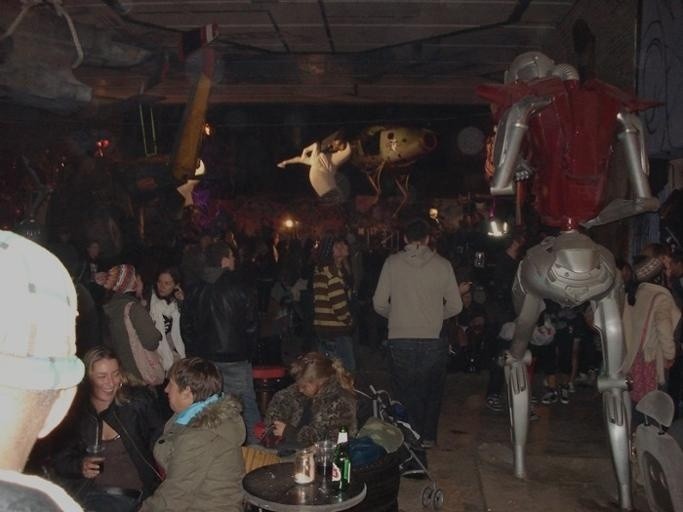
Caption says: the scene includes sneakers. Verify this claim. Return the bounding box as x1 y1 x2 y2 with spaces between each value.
542 386 550 405
551 388 558 404
422 440 435 448
560 383 569 404
569 382 576 394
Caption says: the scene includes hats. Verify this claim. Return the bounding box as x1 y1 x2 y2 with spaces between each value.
94 263 147 307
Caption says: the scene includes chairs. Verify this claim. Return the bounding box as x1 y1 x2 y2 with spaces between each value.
636 390 682 512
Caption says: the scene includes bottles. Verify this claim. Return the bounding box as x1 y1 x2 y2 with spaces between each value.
330 426 353 493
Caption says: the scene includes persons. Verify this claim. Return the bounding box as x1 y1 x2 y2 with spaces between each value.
448 290 490 373
624 242 676 430
44 344 163 511
667 249 683 422
80 239 103 300
312 238 356 382
273 352 358 460
571 302 606 394
372 218 463 449
178 242 206 358
474 50 665 511
142 357 246 511
142 263 196 421
260 230 280 274
540 297 574 406
0 230 84 512
97 264 163 388
194 241 262 446
486 228 530 411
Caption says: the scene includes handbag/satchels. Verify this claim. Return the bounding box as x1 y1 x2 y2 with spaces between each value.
123 301 165 386
348 415 405 470
627 291 673 403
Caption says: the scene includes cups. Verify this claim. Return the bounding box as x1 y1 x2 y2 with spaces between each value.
314 440 336 477
87 445 105 476
294 451 317 485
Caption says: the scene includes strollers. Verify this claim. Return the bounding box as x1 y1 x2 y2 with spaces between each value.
348 368 444 511
